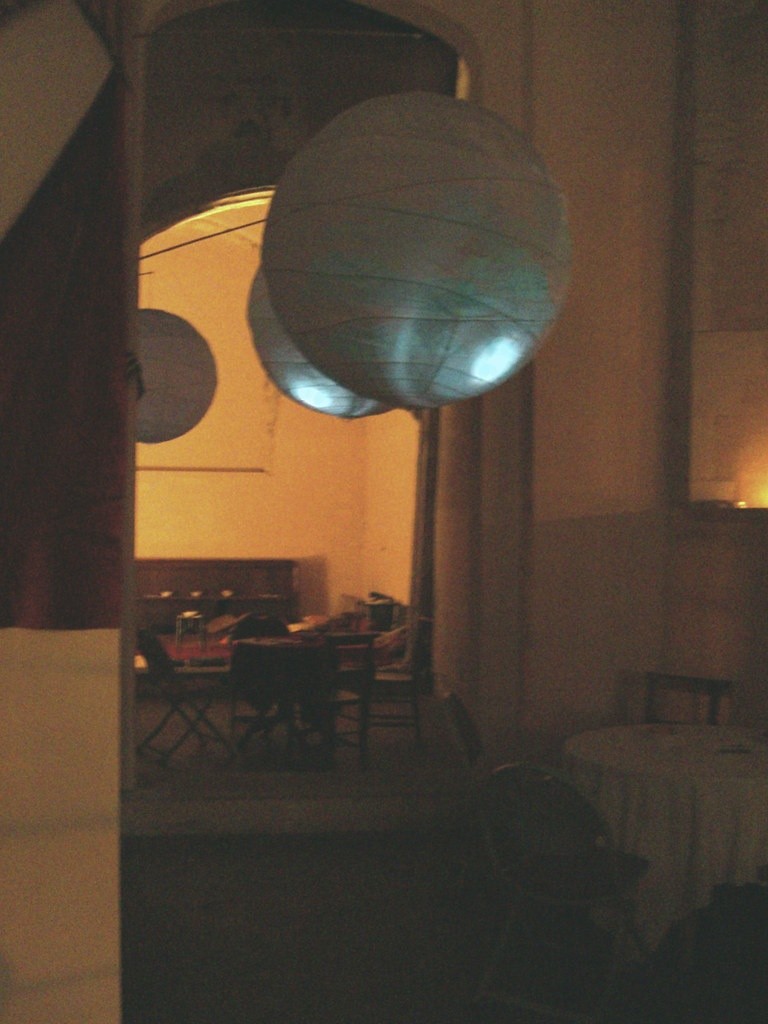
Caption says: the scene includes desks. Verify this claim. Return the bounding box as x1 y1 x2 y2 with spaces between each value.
558 722 767 967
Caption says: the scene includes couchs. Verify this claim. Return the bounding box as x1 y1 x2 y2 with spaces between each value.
133 557 297 637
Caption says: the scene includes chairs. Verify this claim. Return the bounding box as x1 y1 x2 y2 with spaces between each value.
446 673 732 1024
133 593 433 779
